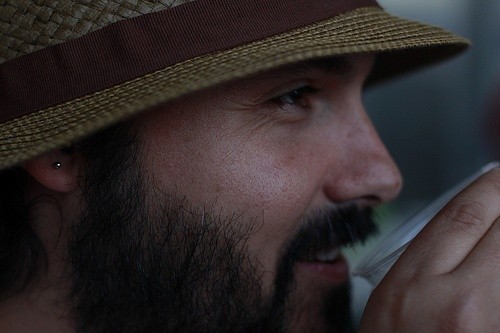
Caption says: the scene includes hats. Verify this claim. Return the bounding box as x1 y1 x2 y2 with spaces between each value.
1 1 471 171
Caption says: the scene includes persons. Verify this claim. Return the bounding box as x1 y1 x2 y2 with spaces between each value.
0 1 500 333
